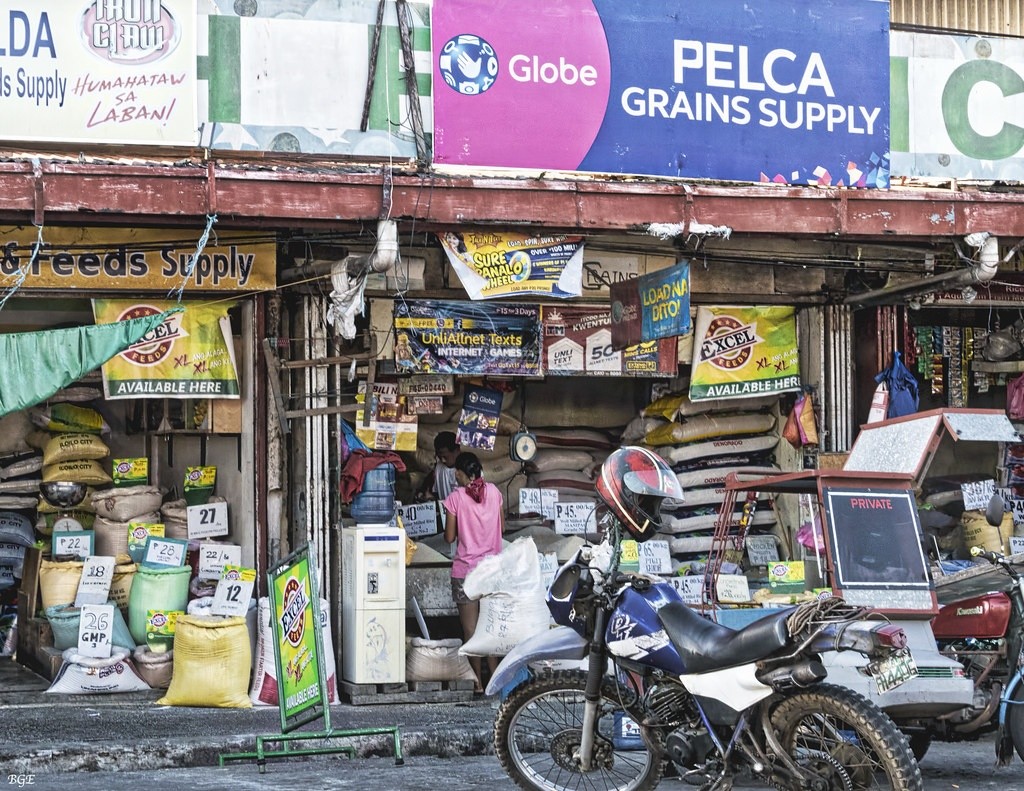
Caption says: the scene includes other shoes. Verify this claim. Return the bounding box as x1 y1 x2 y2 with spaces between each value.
475 688 484 693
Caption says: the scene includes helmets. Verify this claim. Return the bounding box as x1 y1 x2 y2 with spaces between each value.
593 446 677 543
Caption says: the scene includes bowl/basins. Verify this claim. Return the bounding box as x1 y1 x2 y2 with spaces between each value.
39 481 88 508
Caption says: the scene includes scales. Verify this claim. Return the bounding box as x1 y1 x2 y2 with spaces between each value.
503 431 548 527
39 481 88 532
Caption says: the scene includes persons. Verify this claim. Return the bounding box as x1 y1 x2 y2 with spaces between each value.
441 452 507 694
413 431 484 504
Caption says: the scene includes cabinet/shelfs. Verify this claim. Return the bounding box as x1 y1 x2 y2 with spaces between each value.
125 336 243 436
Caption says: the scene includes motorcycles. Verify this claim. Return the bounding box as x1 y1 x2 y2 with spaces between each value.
701 408 1023 772
485 487 925 791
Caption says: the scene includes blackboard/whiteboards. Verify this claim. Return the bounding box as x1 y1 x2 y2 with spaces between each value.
822 487 936 591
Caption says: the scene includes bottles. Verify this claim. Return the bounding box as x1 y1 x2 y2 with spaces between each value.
351 462 396 523
